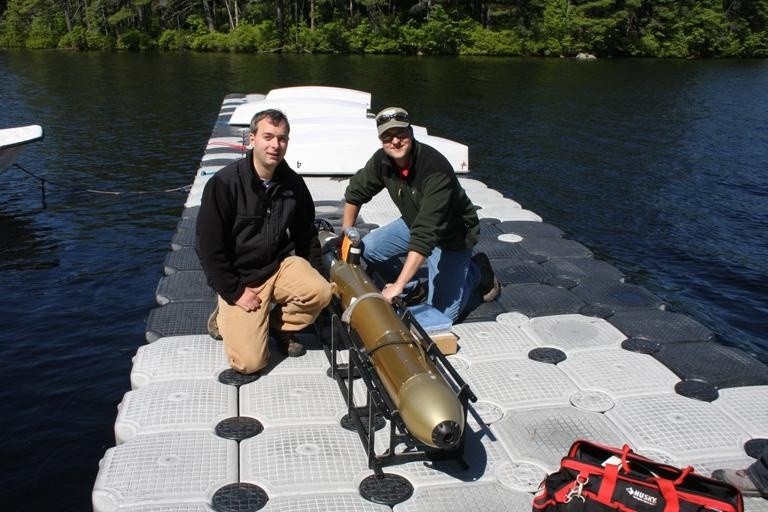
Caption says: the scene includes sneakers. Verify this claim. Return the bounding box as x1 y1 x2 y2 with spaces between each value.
207 303 221 339
473 252 499 301
390 282 426 307
711 467 762 497
269 327 306 360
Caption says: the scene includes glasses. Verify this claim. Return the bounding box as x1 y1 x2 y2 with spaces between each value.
377 113 409 126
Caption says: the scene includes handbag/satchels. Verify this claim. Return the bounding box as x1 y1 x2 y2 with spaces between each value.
534 436 743 512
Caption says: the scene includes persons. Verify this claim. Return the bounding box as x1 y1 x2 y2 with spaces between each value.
337 106 500 325
195 110 333 375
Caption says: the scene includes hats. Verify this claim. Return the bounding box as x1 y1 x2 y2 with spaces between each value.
375 106 410 135
